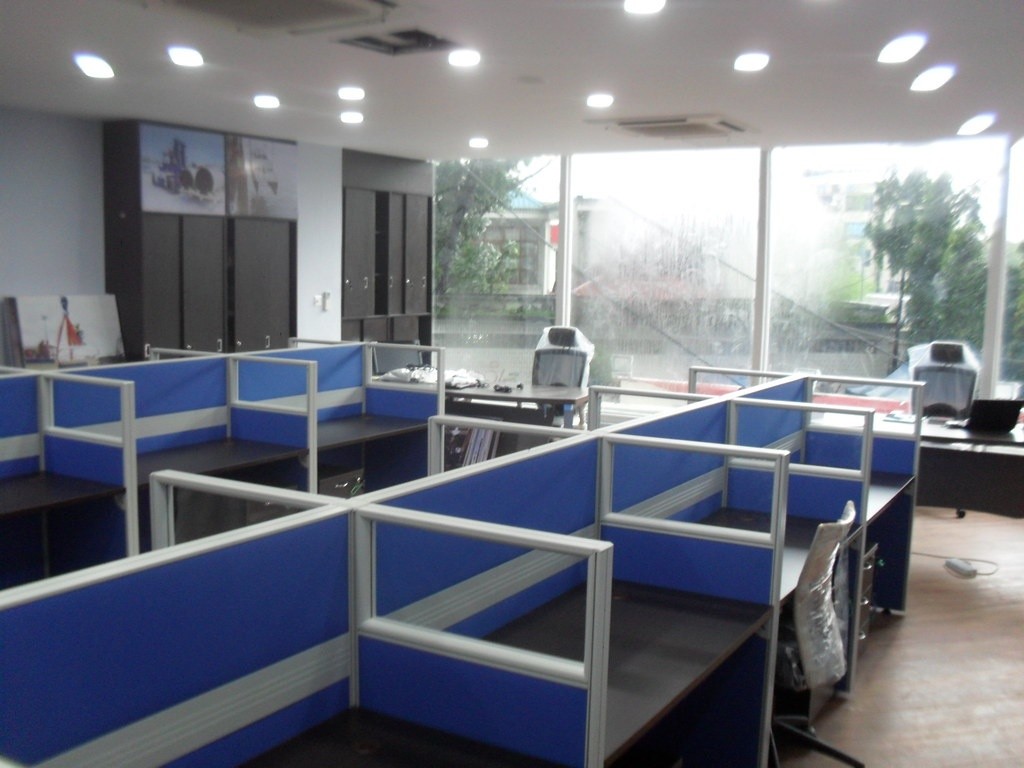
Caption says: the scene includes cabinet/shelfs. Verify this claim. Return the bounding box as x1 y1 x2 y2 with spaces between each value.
342 148 437 366
99 114 301 359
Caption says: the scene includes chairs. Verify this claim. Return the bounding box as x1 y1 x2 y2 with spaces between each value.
910 342 980 518
530 327 593 429
369 339 424 379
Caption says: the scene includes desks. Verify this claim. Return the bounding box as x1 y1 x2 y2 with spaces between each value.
1 393 914 768
368 364 591 430
808 397 1024 456
